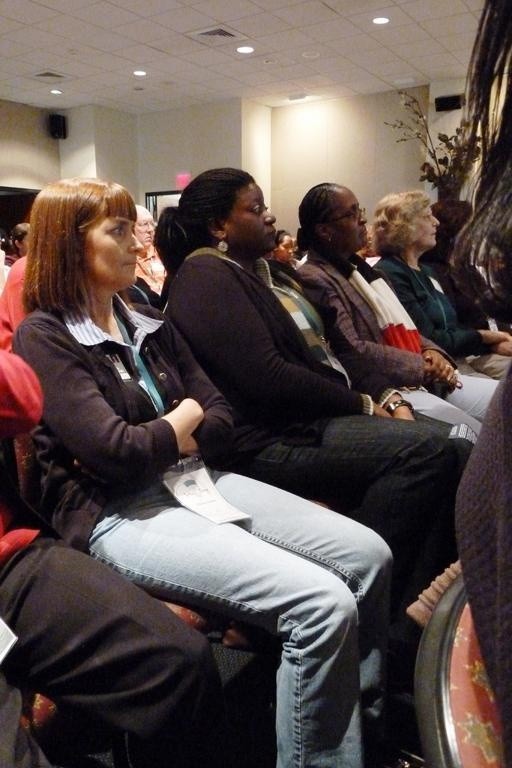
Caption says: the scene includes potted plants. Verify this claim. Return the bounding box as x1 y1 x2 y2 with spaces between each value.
383 88 482 261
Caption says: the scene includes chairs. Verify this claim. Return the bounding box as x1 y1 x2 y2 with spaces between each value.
16 693 54 731
12 429 210 632
397 574 512 766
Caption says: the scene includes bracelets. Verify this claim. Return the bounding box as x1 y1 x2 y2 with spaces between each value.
389 398 414 413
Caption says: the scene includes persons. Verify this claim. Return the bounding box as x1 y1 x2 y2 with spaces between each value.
368 185 512 380
0 350 220 768
359 224 374 261
11 177 397 766
292 183 502 437
0 222 30 350
271 230 296 265
151 167 475 624
130 205 168 310
419 199 511 337
435 1 511 767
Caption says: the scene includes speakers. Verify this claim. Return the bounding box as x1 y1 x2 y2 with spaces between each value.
436 94 468 111
47 113 67 140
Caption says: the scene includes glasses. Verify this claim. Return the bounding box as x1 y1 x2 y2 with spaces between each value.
330 207 368 224
